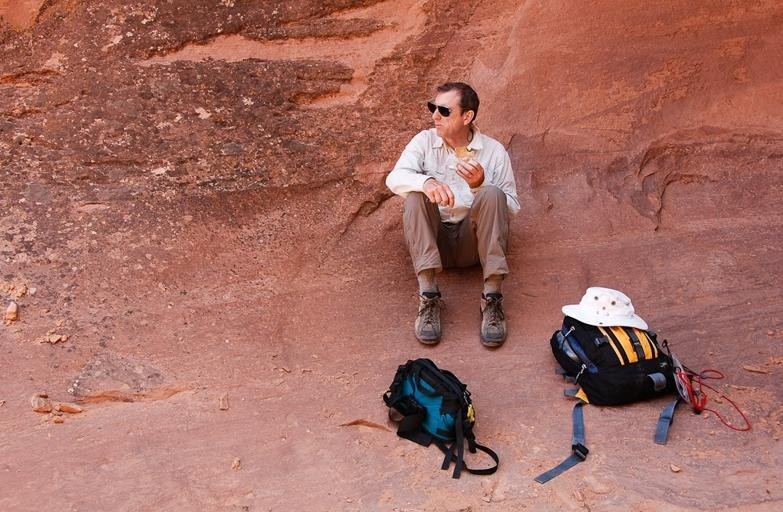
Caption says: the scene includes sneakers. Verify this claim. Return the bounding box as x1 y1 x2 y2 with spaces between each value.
414 290 442 345
479 291 507 348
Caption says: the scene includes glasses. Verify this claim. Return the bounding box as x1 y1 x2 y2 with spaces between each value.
427 100 472 118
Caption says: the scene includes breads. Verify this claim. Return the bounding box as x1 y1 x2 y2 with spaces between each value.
455 146 477 157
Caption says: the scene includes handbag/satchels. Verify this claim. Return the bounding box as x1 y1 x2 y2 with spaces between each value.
384 359 476 443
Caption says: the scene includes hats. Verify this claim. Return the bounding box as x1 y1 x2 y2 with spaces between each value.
560 286 648 330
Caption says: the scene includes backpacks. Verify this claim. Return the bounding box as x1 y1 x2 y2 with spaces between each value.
550 313 692 407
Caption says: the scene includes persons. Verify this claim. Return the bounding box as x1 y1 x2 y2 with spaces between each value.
386 82 521 348
